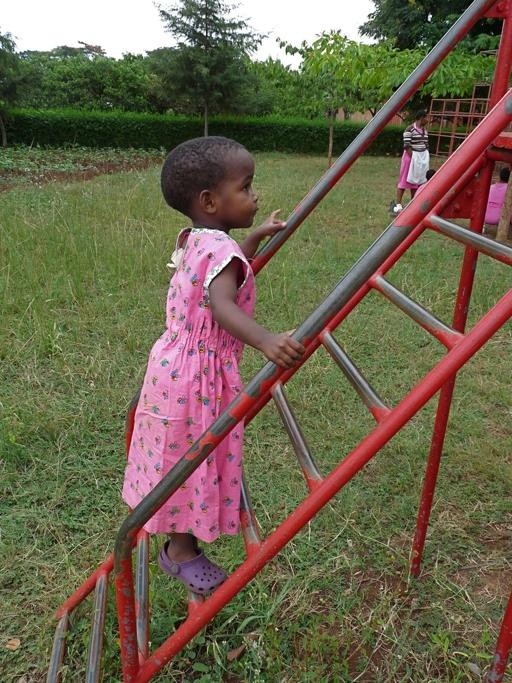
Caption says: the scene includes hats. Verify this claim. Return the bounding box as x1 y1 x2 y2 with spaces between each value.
415 109 428 119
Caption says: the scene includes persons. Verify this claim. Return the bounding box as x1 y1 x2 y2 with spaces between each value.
482 168 512 234
417 168 435 189
121 136 307 595
396 109 430 211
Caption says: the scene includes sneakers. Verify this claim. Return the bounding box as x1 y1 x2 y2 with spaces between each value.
396 204 402 210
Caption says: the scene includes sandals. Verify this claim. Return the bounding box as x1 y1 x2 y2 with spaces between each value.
158 540 226 594
